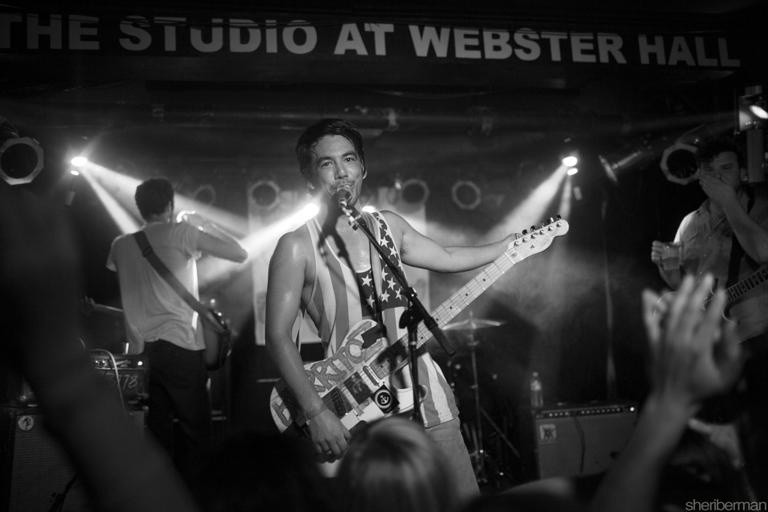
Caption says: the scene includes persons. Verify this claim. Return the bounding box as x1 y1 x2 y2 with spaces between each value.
582 269 766 510
0 189 195 511
651 132 768 510
106 177 248 510
264 118 525 511
328 414 577 509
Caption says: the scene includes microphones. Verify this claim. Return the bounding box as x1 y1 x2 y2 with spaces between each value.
335 184 353 209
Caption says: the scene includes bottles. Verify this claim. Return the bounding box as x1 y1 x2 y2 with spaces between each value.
528 371 545 410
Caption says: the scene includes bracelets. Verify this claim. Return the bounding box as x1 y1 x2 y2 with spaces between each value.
304 402 328 421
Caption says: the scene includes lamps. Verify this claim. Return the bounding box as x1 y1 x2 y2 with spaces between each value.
172 175 216 205
247 178 282 209
0 117 44 186
399 178 430 207
451 179 482 210
559 93 768 186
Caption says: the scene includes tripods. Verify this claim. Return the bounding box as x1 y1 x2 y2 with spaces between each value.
457 329 519 482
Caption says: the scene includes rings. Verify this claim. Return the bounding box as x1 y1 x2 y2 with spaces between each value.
324 447 332 454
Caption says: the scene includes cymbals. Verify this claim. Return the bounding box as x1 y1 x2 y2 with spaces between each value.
440 317 501 330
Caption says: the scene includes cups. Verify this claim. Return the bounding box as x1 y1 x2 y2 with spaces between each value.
659 242 681 272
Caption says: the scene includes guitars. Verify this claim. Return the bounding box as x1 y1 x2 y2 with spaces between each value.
653 264 768 331
76 296 230 370
270 213 570 478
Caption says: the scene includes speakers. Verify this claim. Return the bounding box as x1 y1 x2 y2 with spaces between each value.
520 401 641 478
